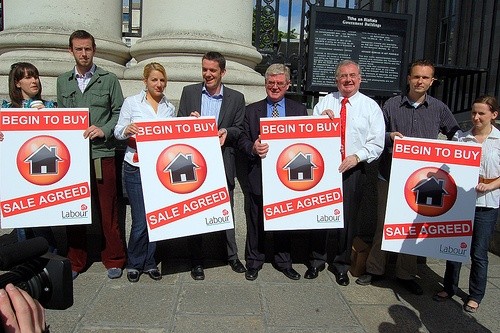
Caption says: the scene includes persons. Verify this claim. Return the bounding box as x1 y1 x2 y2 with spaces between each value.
432 95 500 312
114 61 175 283
239 64 309 280
0 61 57 239
177 52 246 280
55 30 127 279
355 59 460 297
302 60 385 286
0 283 47 333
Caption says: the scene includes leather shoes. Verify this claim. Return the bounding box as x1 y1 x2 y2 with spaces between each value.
304 263 325 279
191 264 204 280
397 276 423 295
357 273 385 284
245 265 262 280
282 267 301 279
336 271 349 285
230 258 246 272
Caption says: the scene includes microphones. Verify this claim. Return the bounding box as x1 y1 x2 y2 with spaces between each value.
0 236 49 271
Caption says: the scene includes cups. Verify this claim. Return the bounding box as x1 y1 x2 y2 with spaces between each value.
30 100 43 108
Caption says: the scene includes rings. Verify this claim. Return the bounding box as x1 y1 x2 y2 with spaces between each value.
90 132 94 135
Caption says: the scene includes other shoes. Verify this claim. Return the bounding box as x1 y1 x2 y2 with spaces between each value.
433 291 453 300
465 299 479 312
127 271 138 283
108 268 122 279
149 270 162 281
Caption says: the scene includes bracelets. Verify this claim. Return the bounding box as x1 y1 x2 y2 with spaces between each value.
353 154 361 164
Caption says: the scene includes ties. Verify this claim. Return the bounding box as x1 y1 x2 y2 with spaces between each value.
340 98 348 162
272 103 279 118
